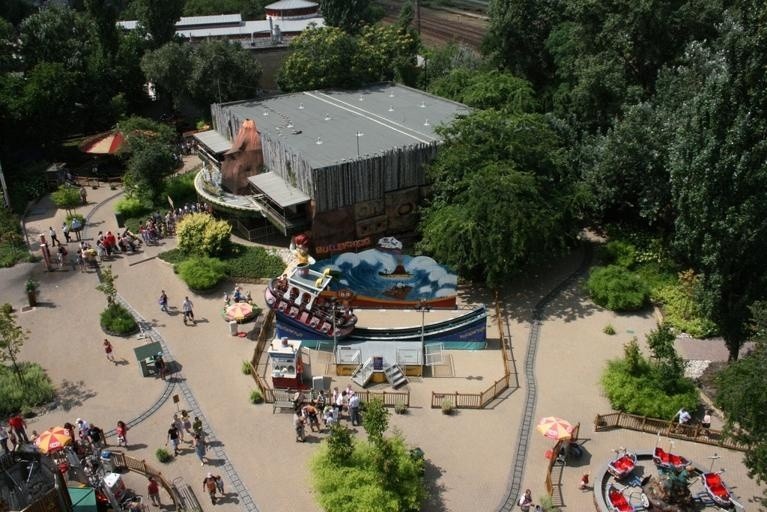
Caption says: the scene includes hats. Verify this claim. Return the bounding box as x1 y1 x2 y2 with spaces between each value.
75 418 80 424
207 473 213 479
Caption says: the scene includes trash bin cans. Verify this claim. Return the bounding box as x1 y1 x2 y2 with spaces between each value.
100 452 115 473
230 321 237 336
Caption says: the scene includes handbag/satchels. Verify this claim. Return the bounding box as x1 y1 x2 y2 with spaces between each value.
117 435 125 443
159 299 165 305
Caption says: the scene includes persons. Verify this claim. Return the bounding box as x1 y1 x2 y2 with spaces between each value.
104 339 113 361
578 473 591 490
80 185 87 202
155 352 167 380
290 233 309 263
160 290 169 314
49 202 212 273
672 408 710 428
519 489 543 512
0 411 224 509
177 137 197 154
224 287 252 306
183 296 194 326
294 384 360 442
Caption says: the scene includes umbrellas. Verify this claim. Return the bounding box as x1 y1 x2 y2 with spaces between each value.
534 416 577 441
78 123 160 154
